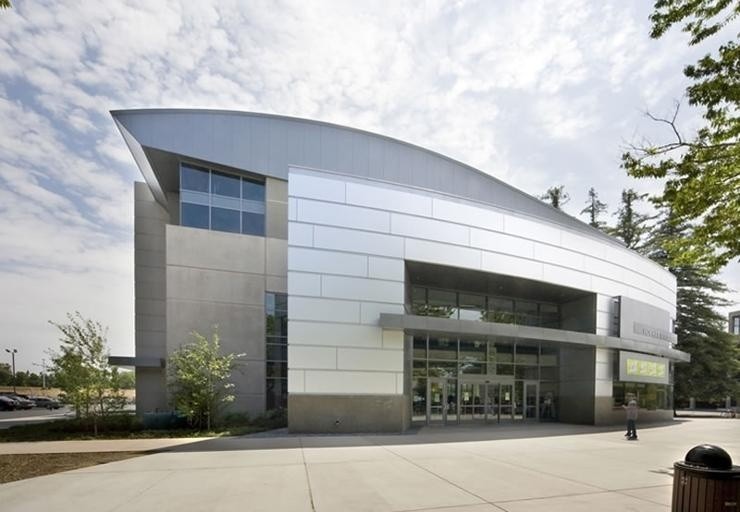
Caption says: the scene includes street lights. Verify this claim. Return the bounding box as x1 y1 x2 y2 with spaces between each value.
4 349 17 393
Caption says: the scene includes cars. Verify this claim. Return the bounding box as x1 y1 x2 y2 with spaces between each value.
0 392 63 411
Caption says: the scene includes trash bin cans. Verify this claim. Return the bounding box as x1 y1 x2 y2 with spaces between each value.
672 444 740 511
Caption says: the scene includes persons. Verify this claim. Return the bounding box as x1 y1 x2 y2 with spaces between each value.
622 393 638 440
